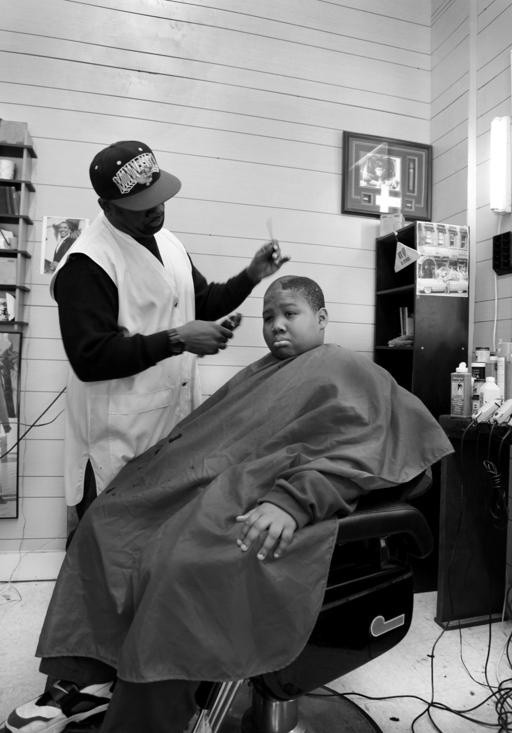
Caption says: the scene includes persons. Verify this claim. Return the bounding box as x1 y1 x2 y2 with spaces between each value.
48 141 290 536
2 278 408 729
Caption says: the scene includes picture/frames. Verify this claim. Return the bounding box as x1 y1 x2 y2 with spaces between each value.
341 128 432 221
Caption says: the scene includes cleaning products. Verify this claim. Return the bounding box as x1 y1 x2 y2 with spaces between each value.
471 339 512 415
450 362 471 417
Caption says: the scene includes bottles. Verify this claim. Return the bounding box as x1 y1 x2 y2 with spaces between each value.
449 345 499 419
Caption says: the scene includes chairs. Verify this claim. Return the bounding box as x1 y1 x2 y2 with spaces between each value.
184 457 437 733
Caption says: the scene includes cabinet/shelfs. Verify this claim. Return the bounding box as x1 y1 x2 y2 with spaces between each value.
0 118 38 327
373 222 469 420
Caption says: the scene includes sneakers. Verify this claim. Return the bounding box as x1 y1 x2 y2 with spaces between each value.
4 673 117 733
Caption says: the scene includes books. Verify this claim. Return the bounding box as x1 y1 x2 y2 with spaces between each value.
389 301 415 347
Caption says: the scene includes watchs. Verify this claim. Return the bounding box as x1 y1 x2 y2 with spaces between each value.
166 327 185 356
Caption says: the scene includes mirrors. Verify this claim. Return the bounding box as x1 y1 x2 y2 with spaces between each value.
0 333 19 518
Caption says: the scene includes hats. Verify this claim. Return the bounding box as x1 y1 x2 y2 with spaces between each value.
89 139 182 213
